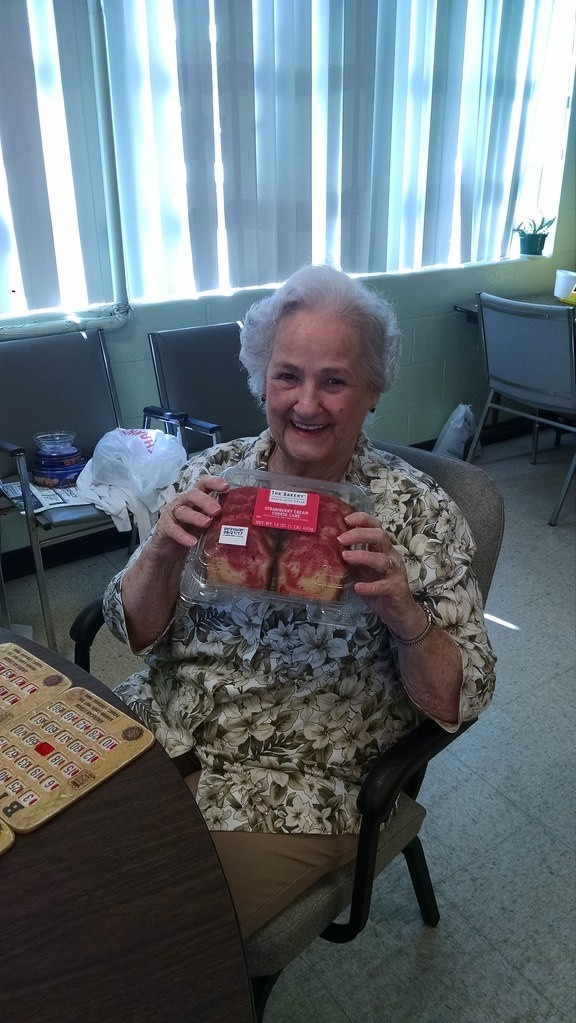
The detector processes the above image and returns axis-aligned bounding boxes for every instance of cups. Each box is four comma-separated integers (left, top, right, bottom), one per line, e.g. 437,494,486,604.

553,269,576,299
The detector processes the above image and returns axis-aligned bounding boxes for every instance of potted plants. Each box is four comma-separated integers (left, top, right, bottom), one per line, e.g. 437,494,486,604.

512,213,556,254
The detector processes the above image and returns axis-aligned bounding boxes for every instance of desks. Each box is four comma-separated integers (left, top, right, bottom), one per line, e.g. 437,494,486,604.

452,291,575,464
0,625,264,1023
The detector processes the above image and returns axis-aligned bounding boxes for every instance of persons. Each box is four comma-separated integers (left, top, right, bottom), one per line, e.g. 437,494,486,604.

103,263,497,946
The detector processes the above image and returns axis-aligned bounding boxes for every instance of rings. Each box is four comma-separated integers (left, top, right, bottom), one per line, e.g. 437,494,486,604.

378,553,395,578
170,504,186,523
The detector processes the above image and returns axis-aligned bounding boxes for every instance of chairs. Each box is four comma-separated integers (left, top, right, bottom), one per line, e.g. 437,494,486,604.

56,430,508,1023
465,293,576,526
147,318,271,459
0,326,188,655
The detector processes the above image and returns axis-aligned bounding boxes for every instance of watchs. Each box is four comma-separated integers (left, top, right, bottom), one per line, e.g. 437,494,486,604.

388,603,438,647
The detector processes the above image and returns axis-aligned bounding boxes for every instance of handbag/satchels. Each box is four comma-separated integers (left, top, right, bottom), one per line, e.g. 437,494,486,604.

434,403,481,462
93,428,187,502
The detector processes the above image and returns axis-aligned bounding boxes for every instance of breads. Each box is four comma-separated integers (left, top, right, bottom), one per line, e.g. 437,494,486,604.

205,486,359,600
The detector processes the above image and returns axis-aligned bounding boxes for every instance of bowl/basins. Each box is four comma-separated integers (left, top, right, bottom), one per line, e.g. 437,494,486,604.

32,429,76,454
177,466,376,633
